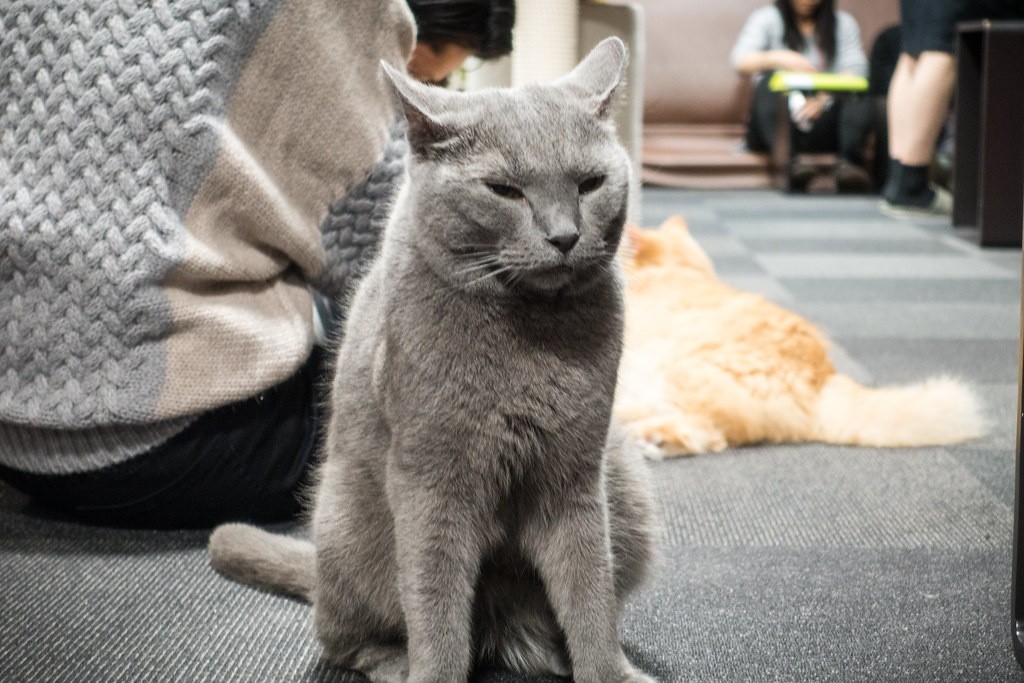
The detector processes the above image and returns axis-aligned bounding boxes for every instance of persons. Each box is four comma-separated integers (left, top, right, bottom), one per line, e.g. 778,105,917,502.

0,0,517,531
732,0,961,216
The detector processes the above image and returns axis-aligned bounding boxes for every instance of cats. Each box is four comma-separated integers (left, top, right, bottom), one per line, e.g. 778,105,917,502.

207,36,656,683
609,210,992,463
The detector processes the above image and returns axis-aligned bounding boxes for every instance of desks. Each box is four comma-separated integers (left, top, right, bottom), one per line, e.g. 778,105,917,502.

952,16,1024,247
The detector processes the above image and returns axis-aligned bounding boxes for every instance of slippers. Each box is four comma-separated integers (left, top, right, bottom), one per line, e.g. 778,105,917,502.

875,177,953,223
